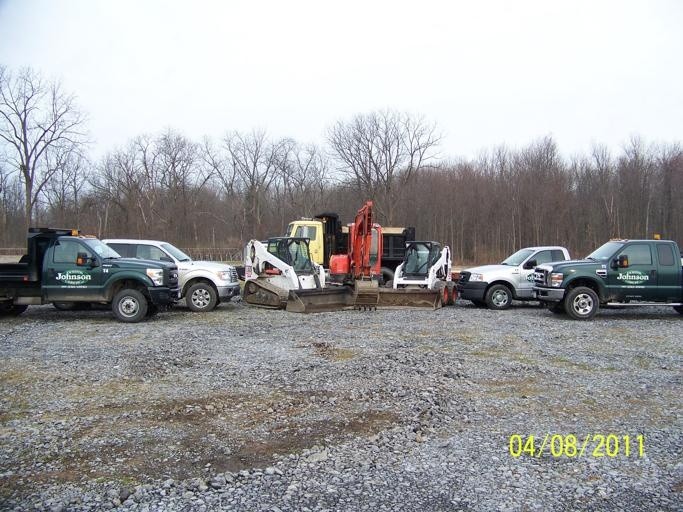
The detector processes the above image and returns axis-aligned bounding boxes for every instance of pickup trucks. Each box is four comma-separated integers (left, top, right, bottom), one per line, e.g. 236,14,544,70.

0,201,242,323
453,234,683,319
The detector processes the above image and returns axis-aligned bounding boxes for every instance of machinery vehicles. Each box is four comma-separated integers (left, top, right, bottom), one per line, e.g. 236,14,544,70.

234,201,458,314
